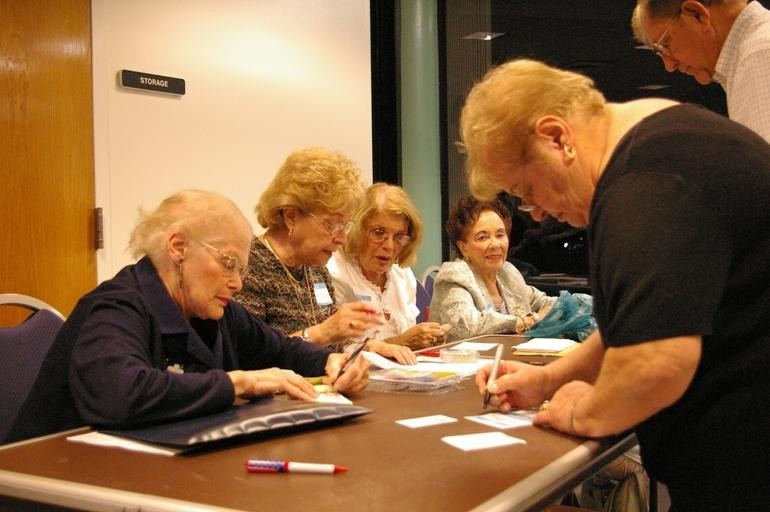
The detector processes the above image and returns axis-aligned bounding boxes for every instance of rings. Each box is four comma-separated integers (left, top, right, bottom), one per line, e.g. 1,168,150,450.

348,320,356,328
540,400,550,412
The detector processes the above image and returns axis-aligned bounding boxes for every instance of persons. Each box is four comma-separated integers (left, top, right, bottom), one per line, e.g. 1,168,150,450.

457,58,770,512
0,191,370,446
228,149,419,366
427,197,559,342
326,183,453,351
631,0,770,147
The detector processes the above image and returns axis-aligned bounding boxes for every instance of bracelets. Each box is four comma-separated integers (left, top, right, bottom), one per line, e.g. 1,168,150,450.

566,396,579,438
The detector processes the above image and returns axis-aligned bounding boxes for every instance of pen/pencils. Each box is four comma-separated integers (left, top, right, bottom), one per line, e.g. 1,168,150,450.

483,343,505,409
418,351,439,357
244,459,349,474
331,337,371,385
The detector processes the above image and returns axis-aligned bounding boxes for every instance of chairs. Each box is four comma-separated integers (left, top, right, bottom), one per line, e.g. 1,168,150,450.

417,280,433,323
0,293,68,447
421,266,441,297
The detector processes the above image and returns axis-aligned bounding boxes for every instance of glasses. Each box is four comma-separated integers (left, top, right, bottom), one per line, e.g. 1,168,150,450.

361,225,413,247
518,128,537,212
653,9,683,54
192,235,251,282
300,209,353,238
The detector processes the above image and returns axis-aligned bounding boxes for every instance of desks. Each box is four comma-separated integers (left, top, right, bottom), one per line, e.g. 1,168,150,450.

1,334,659,512
